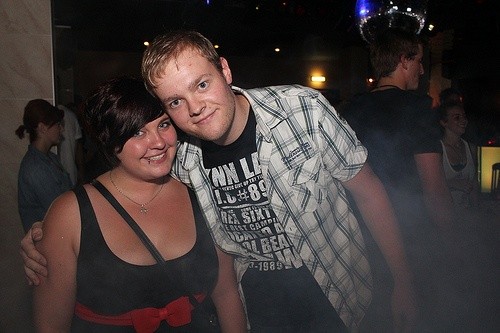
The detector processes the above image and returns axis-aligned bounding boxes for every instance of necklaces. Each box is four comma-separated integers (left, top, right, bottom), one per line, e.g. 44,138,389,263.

110,167,165,214
445,141,465,163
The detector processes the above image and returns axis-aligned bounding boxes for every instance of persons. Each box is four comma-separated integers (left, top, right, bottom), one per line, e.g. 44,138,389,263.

140,27,418,333
17,99,110,235
18,73,247,333
321,11,500,333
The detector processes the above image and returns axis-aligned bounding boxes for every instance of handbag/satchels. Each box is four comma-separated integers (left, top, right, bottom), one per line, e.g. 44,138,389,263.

193,307,220,332
